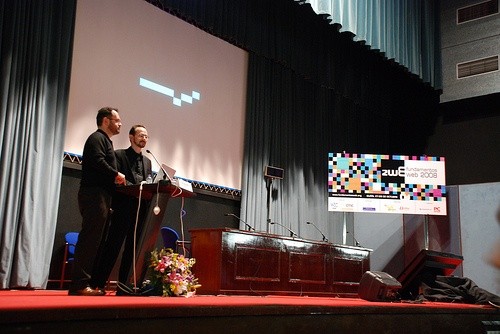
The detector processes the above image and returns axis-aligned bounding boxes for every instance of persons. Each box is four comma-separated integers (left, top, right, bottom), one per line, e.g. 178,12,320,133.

90,124,153,295
67,107,126,296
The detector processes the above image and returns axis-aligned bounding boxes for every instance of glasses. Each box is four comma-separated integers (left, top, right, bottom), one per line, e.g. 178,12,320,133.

135,135,150,140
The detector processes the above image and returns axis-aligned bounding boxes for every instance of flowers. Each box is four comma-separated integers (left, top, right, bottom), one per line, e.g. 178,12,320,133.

141,249,201,298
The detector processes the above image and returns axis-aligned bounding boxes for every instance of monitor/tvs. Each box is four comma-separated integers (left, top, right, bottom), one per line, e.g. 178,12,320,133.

395,250,465,301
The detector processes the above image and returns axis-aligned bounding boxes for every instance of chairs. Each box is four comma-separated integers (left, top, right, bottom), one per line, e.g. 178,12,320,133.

161,226,182,248
59,231,82,290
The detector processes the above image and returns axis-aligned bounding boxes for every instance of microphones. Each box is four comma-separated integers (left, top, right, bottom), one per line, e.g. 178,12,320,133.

307,222,330,242
146,150,173,185
271,222,297,236
225,213,256,231
347,232,361,247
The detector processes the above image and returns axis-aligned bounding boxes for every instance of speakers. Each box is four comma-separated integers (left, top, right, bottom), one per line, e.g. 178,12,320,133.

359,271,402,302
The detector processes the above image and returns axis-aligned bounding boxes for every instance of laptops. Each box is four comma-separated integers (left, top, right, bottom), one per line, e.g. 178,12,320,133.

152,164,176,183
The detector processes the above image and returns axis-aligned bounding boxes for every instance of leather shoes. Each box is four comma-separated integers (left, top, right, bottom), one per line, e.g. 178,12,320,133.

67,286,104,296
